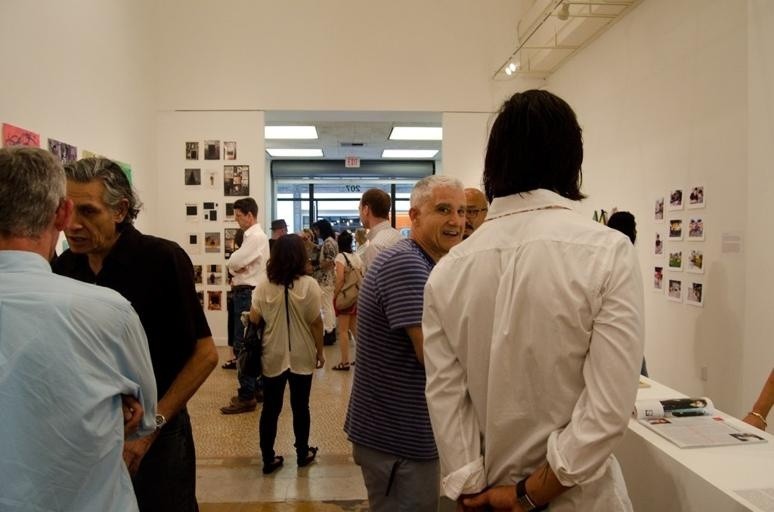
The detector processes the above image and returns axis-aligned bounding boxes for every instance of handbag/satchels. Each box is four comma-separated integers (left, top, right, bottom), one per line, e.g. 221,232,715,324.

235,316,266,378
336,251,365,310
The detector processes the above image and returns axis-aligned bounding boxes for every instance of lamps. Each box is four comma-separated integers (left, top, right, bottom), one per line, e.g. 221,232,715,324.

505,62,520,76
558,0,569,20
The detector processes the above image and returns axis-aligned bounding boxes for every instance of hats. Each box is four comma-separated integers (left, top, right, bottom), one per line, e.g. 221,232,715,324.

270,220,288,230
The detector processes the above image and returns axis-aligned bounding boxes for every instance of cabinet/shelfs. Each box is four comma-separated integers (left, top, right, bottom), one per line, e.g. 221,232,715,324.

303,216,364,251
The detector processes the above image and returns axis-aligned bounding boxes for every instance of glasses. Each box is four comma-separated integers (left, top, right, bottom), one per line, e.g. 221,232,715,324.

466,208,487,218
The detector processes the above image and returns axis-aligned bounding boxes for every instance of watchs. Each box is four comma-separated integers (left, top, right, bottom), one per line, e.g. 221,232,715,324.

151,412,166,429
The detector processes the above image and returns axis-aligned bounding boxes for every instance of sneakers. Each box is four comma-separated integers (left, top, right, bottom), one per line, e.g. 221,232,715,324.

220,389,263,414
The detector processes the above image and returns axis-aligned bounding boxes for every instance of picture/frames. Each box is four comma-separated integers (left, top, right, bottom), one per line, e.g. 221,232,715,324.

223,165,249,196
224,228,244,259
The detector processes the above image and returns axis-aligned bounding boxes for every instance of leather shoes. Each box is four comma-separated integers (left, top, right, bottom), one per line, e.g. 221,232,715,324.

264,456,283,474
297,448,316,467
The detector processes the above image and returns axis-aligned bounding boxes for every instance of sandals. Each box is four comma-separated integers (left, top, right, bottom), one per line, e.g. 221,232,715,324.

222,359,236,368
332,361,349,371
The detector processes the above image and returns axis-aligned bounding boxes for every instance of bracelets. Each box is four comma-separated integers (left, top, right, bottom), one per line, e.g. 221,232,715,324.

515,477,550,509
747,411,768,428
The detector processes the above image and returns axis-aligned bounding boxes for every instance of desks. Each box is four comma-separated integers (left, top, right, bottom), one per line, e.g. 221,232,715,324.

612,374,774,512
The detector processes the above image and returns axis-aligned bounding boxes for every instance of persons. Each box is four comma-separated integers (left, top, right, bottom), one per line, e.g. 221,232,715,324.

0,147,153,511
311,189,405,372
604,210,649,378
51,157,220,511
460,188,488,238
424,88,650,512
342,171,469,512
663,395,708,411
739,367,774,431
221,197,327,479
